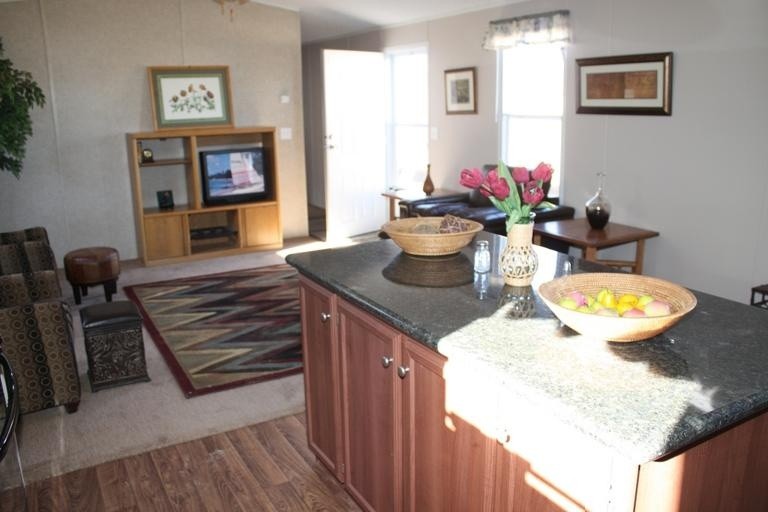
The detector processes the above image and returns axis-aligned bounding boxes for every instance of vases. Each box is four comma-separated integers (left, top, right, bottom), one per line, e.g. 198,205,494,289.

497,221,536,287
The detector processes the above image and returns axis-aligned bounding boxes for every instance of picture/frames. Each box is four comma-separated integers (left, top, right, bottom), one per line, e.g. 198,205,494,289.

443,66,476,115
573,51,671,118
145,63,234,132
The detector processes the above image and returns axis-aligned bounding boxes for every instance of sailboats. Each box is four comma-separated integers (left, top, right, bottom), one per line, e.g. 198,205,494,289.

227,152,262,189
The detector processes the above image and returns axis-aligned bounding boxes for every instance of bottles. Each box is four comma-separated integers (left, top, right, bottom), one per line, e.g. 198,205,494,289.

585,187,612,230
471,274,491,300
472,240,491,273
423,164,434,194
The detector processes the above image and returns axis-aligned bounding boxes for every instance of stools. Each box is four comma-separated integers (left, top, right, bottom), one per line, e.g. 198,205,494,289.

64,246,121,305
79,299,150,393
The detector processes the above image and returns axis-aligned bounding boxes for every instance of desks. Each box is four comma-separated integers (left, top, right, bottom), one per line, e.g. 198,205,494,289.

531,217,660,273
398,194,576,256
285,221,768,512
379,187,460,221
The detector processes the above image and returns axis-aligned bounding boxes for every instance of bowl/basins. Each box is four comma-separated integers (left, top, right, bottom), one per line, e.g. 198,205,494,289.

537,272,698,342
381,250,475,287
384,216,485,256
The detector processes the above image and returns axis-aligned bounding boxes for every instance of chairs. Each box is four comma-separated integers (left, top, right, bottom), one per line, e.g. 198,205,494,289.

0,270,80,417
0,227,57,276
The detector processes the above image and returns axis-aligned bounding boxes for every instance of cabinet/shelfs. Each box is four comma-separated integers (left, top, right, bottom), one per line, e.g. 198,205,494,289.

125,126,282,268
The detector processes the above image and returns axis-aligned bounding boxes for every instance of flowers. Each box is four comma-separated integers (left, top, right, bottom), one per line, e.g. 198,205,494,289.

457,164,553,228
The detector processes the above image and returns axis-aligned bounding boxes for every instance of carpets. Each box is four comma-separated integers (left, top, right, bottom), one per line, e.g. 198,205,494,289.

0,230,398,494
121,261,301,401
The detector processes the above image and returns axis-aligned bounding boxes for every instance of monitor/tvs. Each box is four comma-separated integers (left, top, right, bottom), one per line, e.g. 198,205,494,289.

199,146,267,205
